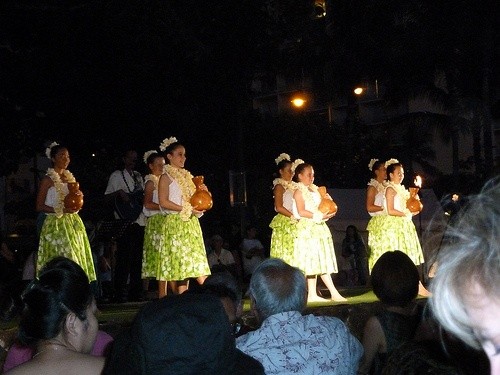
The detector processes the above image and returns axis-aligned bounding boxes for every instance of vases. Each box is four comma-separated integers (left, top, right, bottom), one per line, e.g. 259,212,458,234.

318,186,338,214
65,181,84,213
407,188,424,212
191,175,213,210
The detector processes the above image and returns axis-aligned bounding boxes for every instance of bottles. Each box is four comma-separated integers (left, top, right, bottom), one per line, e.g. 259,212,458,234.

406,188,423,212
190,176,213,211
64,183,83,211
317,186,337,215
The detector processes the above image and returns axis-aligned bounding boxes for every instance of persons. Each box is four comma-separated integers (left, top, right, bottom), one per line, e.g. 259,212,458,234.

201,285,249,337
358,251,429,375
0,267,111,375
242,227,266,297
4,257,113,371
350,241,365,287
422,177,500,375
204,272,244,317
158,137,212,293
36,142,100,316
102,291,264,375
142,150,178,298
342,225,368,283
346,307,369,341
235,258,365,375
105,150,147,302
380,158,431,297
382,341,469,375
290,158,347,302
208,235,236,270
304,301,365,322
0,152,140,297
201,237,233,254
0,273,15,375
366,158,389,276
270,153,299,266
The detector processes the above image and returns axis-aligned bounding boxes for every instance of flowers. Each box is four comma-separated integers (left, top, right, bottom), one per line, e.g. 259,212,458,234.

274,153,291,166
384,159,398,168
290,158,304,171
159,137,177,150
163,164,196,200
144,173,160,188
273,178,297,189
368,178,384,202
314,210,325,224
143,150,157,163
404,211,415,223
45,142,58,158
179,202,193,221
368,158,379,172
287,181,332,209
47,168,80,203
385,181,409,210
53,203,64,220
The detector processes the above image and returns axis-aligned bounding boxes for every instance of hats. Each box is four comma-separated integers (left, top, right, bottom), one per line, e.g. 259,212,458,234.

211,234,223,240
